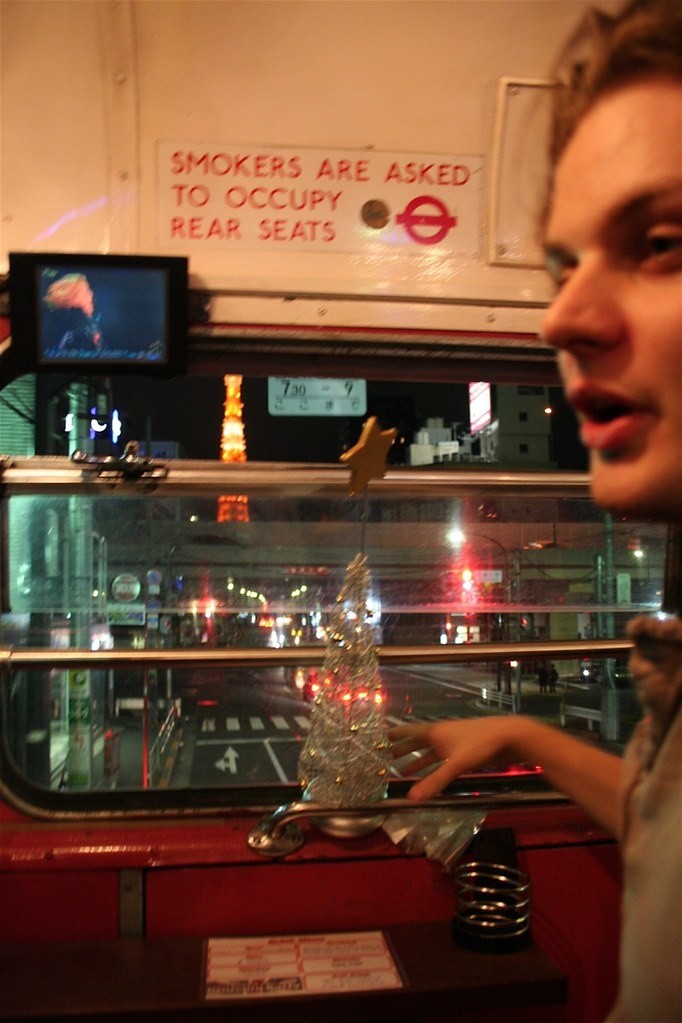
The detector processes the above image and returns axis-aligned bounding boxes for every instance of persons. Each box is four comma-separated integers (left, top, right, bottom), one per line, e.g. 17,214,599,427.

384,3,682,1023
43,271,104,350
537,662,559,693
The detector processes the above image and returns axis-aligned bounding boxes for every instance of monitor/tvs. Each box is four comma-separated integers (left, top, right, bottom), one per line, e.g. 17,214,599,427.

9,253,190,374
